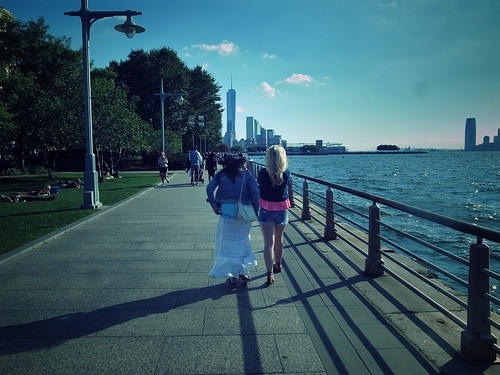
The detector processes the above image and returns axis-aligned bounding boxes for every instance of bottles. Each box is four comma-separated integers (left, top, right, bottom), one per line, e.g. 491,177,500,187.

15,196,19,203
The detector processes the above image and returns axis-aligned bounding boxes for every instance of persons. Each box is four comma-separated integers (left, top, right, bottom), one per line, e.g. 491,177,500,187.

256,144,294,284
200,151,212,170
206,153,261,282
188,150,192,172
32,185,51,195
216,151,244,169
190,145,202,186
112,171,124,179
57,177,82,188
104,172,114,179
206,152,216,182
159,152,170,187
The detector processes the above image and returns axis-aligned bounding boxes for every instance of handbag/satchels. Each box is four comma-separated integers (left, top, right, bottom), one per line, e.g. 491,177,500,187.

238,202,257,222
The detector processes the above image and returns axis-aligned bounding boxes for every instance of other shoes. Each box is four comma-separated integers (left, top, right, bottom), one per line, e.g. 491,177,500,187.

273,262,281,272
166,178,169,183
267,271,274,284
229,275,237,284
239,275,250,282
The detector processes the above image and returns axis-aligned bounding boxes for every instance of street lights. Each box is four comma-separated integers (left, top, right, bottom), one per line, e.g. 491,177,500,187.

153,79,189,152
64,0,147,208
